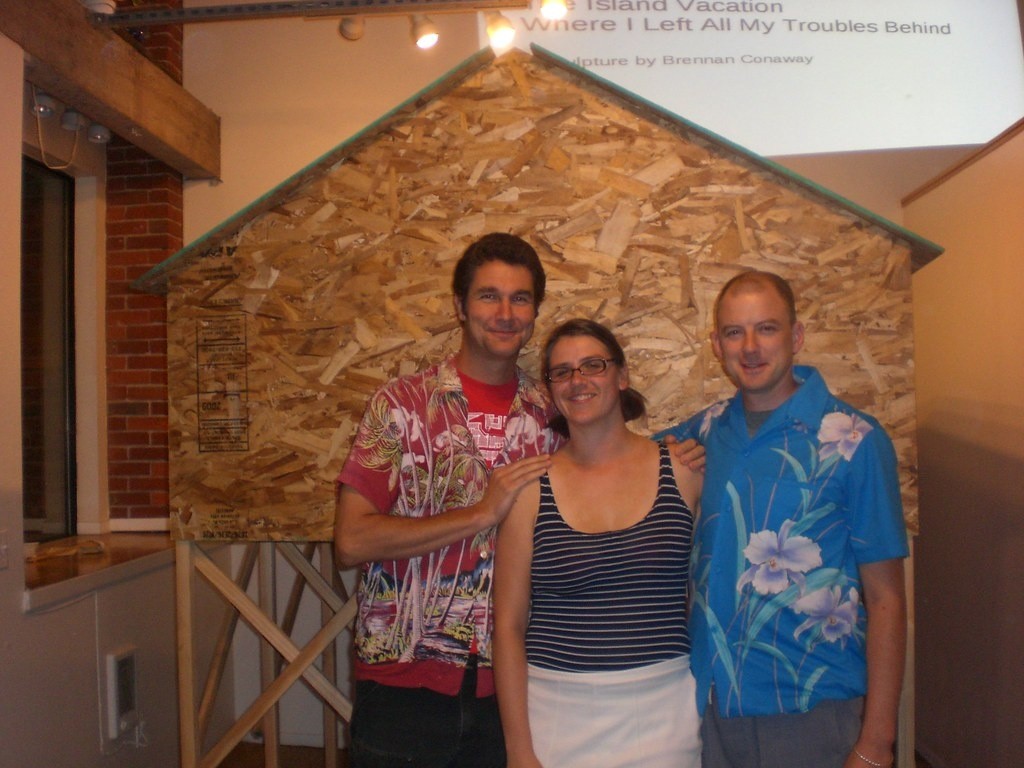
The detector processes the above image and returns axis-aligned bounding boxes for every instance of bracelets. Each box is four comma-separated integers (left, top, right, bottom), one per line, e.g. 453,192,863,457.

853,744,894,767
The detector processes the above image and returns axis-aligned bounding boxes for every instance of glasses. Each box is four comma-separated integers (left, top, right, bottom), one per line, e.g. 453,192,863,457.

545,357,616,382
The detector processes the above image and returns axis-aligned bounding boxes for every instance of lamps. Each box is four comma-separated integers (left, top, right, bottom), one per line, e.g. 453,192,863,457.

483,11,515,44
410,15,439,49
338,18,364,41
540,0,568,17
88,122,110,143
60,108,83,131
30,88,54,118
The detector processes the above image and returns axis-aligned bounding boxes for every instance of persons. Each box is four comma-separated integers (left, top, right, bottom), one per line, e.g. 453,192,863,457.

333,232,705,768
493,315,704,768
646,271,910,768
